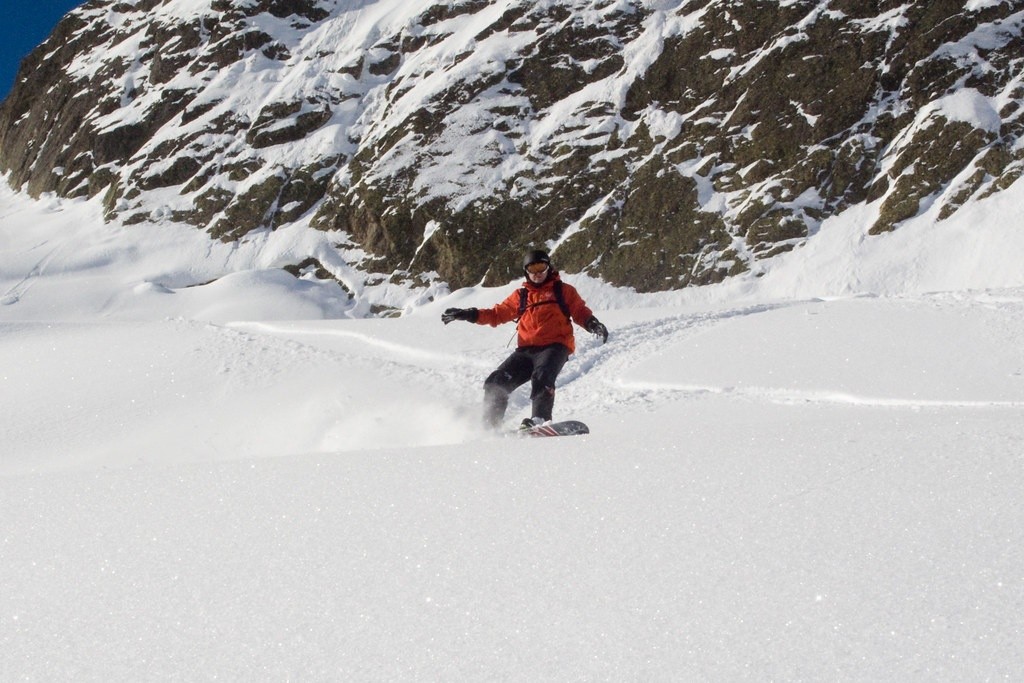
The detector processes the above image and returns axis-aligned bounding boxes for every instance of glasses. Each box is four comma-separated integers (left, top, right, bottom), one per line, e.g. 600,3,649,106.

526,265,549,276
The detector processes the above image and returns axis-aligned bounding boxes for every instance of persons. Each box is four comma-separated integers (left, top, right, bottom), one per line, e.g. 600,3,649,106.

441,251,609,428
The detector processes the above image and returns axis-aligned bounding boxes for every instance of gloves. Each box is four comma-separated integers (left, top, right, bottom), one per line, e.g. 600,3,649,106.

584,316,608,344
441,307,477,325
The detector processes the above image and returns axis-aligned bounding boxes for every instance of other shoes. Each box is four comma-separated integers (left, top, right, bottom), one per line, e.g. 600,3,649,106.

522,418,537,428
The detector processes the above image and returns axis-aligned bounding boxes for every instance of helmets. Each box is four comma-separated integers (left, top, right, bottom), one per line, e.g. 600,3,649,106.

523,250,550,268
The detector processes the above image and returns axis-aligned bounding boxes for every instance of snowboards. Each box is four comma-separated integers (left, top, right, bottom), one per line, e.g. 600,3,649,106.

517,419,590,437
